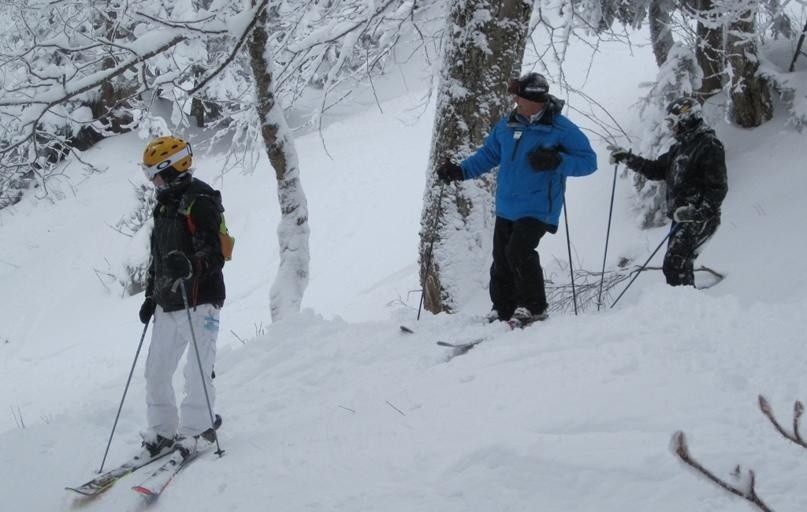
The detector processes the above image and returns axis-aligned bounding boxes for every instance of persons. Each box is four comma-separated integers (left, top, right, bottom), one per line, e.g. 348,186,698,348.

434,72,598,328
135,135,227,460
604,96,729,287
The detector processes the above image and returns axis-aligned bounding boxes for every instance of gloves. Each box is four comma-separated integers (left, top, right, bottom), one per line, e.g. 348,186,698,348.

163,254,187,278
139,295,154,324
436,160,461,184
532,146,558,172
607,144,637,168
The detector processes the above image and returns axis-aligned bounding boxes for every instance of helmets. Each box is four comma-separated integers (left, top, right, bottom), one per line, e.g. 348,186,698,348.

144,136,193,172
664,97,703,130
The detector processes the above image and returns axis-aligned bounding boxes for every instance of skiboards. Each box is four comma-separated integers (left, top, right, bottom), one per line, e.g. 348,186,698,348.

400,304,548,347
64,415,222,496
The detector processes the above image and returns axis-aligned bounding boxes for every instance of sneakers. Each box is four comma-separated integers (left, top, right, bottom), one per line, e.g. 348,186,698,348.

141,431,216,457
489,304,532,318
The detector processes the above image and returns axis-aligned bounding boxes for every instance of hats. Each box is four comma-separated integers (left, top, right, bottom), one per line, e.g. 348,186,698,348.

508,72,549,102
